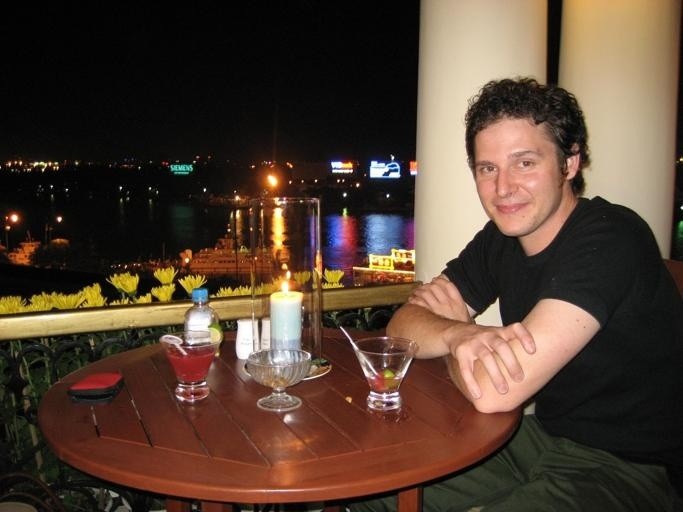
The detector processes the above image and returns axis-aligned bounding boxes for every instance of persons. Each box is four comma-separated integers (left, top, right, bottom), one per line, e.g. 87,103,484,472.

343,72,683,510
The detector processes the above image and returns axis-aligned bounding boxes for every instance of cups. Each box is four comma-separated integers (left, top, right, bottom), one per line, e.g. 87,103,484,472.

159,330,222,402
248,194,323,371
353,337,418,413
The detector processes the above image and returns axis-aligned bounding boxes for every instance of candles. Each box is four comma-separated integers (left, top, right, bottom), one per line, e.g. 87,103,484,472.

270,280,303,349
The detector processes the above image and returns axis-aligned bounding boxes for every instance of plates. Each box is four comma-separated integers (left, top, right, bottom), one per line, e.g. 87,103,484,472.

241,357,332,382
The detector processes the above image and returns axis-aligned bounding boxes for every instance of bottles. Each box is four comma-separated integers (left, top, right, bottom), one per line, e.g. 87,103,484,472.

262,316,271,349
184,288,220,357
235,318,260,361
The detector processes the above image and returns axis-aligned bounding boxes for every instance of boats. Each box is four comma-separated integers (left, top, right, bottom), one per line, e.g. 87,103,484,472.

5,232,70,271
347,245,415,283
103,255,180,276
179,238,291,283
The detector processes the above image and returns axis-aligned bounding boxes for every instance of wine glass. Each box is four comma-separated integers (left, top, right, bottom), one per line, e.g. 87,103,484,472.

249,348,311,413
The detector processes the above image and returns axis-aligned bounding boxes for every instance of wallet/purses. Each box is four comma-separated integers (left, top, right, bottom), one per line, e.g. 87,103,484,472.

66,372,123,405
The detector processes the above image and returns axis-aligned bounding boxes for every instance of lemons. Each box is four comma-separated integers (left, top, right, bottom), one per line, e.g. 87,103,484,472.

208,325,223,347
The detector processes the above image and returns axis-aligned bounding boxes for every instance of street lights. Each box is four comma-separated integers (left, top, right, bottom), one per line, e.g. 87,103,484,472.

43,216,64,250
257,175,278,285
2,215,11,253
229,194,241,285
